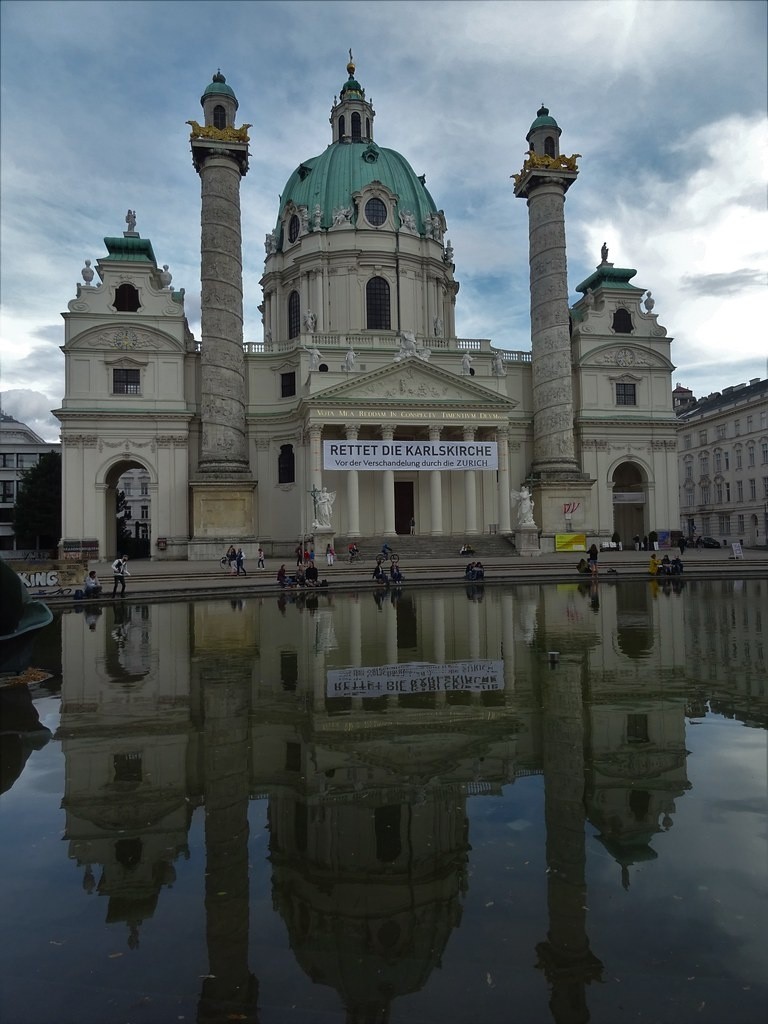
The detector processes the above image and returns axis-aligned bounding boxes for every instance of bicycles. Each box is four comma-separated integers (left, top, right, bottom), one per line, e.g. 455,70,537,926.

376,551,399,564
220,557,232,569
343,550,365,565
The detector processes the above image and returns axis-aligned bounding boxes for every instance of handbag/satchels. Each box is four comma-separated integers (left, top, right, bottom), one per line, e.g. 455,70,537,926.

330,547,334,555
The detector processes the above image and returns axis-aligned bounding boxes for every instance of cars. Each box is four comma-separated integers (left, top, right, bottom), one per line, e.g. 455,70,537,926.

701,536,720,548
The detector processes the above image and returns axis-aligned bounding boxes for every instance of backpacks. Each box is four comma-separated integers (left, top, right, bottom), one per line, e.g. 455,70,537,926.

305,551,310,560
348,544,353,550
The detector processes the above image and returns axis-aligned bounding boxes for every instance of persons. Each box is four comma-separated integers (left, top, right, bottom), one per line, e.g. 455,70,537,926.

85,555,130,598
85,602,130,648
127,927,143,952
230,586,402,617
586,530,705,577
621,867,631,894
126,209,136,232
459,543,477,557
315,487,337,527
589,579,687,615
264,204,454,263
227,542,406,589
466,584,485,604
409,516,415,536
601,242,609,262
511,486,534,524
465,561,485,581
264,309,507,376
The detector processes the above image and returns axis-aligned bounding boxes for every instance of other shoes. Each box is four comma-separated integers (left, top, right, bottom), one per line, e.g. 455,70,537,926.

244,571,246,576
121,594,125,598
261,568,265,570
328,564,330,566
257,568,260,569
330,564,332,566
349,561,352,564
111,595,115,598
